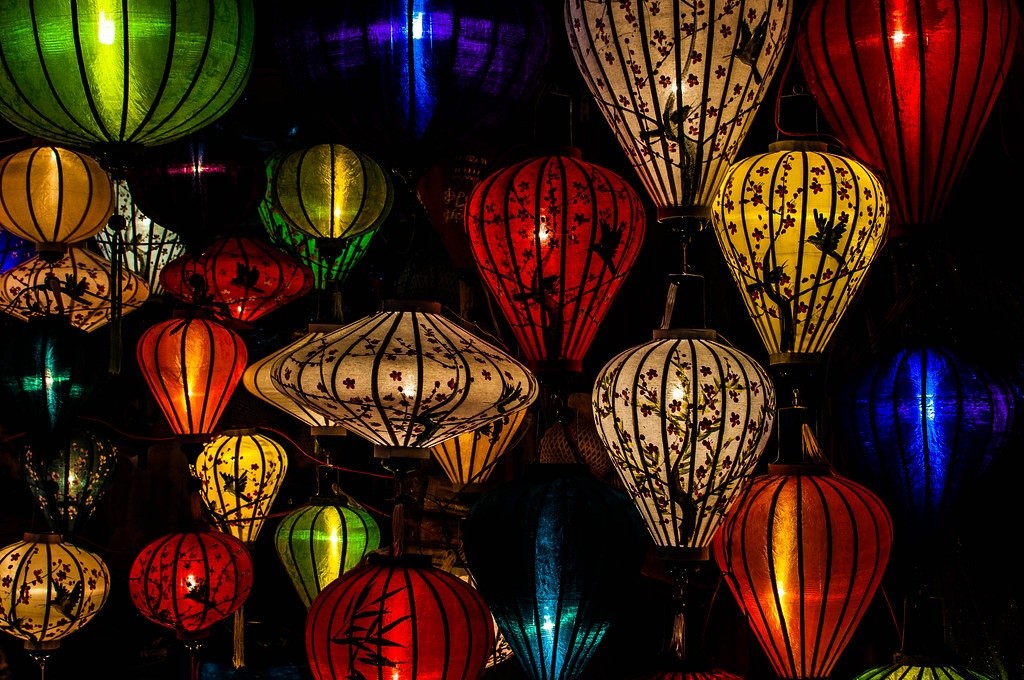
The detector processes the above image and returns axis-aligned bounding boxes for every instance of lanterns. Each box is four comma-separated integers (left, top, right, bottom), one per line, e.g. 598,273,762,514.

0,0,1024,680
